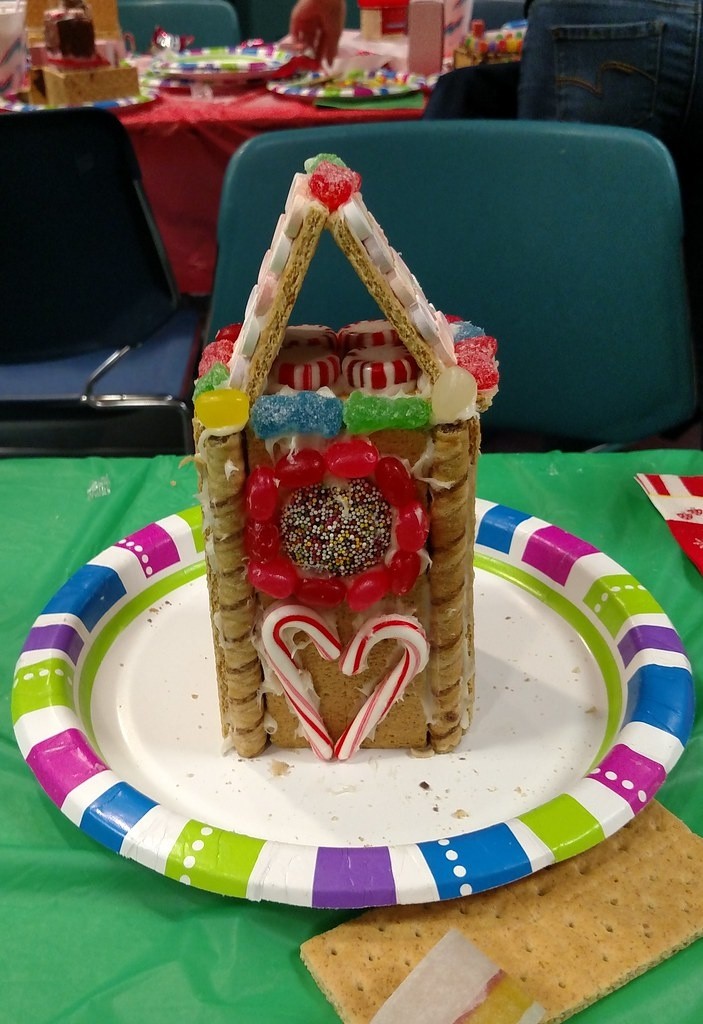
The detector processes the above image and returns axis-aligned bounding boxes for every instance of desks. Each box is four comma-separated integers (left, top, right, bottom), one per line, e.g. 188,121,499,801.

122,94,434,295
0,449,703,1024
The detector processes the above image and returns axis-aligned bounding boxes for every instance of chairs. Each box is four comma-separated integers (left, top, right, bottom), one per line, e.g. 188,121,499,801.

115,0,238,54
203,119,699,451
0,107,203,456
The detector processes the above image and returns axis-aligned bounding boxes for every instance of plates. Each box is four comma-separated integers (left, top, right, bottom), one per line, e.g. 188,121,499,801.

267,72,432,97
10,487,697,907
150,46,293,76
2,82,159,108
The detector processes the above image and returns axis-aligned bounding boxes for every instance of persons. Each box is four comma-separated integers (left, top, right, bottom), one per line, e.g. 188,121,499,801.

289,0,703,205
